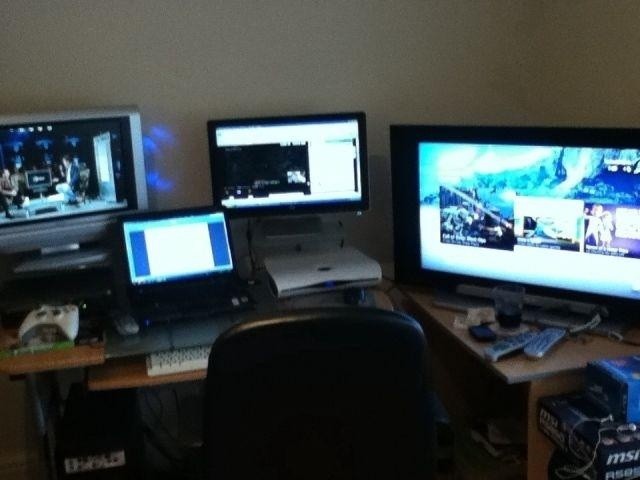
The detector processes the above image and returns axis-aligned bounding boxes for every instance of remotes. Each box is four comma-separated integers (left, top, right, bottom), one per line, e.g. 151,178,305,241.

483,330,540,361
523,327,568,362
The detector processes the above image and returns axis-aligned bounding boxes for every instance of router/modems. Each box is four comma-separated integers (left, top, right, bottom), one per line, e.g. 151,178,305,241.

261,245,384,300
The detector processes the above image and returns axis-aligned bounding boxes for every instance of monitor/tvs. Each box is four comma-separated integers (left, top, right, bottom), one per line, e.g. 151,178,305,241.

205,110,370,238
0,104,152,275
389,123,640,344
115,202,238,290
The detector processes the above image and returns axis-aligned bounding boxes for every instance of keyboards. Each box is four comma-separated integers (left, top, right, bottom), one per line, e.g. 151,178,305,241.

142,344,212,378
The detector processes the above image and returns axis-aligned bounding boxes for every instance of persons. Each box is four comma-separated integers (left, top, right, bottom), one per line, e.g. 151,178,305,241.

583,203,615,250
0,165,25,220
52,155,83,207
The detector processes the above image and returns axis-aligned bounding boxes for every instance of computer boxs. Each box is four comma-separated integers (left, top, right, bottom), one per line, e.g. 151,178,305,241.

52,380,151,480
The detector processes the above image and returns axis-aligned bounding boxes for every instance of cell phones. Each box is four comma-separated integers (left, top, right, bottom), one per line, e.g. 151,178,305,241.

469,323,499,342
